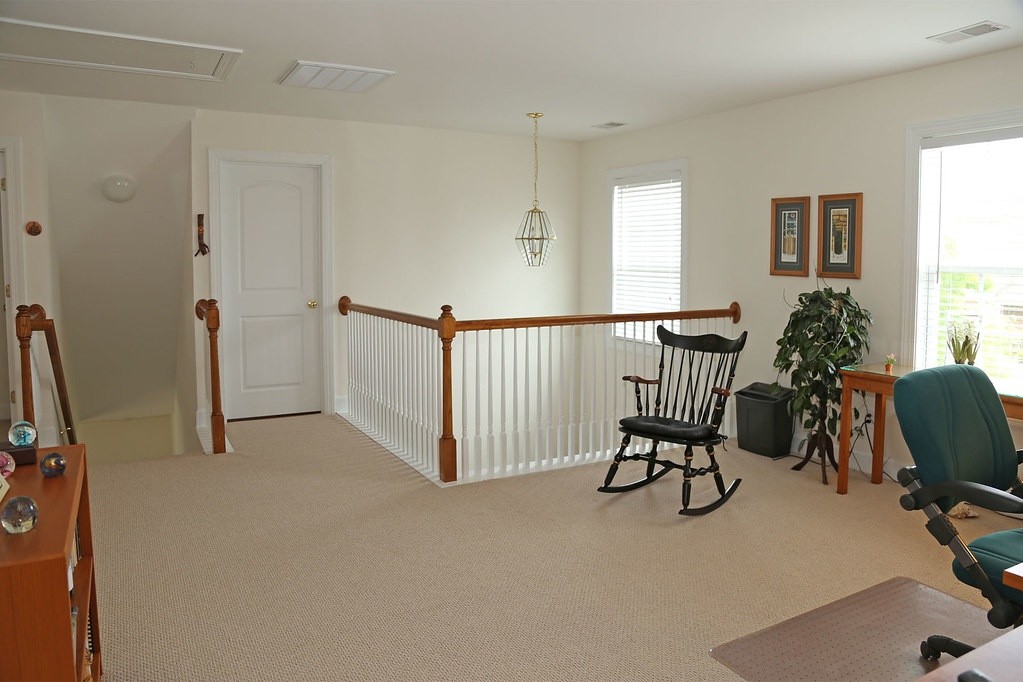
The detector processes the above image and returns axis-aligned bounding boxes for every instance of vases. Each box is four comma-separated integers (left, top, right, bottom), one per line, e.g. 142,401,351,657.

885,364,893,371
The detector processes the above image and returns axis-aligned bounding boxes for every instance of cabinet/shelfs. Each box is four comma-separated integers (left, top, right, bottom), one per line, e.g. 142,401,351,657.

0,444,103,682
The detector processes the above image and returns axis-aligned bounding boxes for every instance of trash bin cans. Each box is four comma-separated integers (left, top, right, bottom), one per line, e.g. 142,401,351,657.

733,381,797,458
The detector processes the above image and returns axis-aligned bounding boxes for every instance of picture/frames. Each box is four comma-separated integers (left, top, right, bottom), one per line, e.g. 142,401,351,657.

817,193,863,280
770,197,810,276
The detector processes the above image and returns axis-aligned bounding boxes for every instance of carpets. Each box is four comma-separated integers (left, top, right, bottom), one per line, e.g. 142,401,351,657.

708,575,1014,682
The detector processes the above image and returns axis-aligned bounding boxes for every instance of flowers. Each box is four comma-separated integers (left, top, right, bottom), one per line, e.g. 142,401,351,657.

885,354,896,364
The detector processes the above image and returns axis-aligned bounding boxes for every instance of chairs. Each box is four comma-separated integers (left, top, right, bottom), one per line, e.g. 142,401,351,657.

598,325,747,515
893,364,1023,659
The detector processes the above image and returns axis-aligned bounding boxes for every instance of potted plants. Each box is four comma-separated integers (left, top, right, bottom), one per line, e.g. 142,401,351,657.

966,332,980,365
772,268,872,457
947,328,975,364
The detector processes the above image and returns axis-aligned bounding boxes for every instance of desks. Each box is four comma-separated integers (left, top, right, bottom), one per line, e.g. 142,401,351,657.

837,362,1023,494
1003,561,1023,591
914,624,1023,682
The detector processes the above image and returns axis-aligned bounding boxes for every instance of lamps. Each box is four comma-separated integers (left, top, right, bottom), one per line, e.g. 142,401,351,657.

104,172,138,203
515,113,556,267
